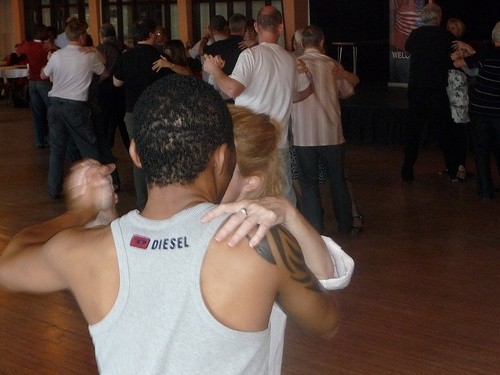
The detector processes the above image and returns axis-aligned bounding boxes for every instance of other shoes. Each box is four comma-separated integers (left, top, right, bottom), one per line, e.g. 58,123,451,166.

35,143,44,148
48,193,59,198
402,166,415,182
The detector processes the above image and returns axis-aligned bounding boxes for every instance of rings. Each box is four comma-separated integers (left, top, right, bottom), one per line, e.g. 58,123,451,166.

239,207,249,219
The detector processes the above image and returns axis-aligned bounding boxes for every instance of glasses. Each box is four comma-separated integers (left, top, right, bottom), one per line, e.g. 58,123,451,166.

154,32,161,36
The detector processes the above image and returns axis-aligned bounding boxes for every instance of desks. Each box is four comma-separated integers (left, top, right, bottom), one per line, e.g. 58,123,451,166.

0,66,28,108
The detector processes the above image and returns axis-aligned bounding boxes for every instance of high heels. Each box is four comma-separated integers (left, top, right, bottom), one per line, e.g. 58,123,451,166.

352,215,366,234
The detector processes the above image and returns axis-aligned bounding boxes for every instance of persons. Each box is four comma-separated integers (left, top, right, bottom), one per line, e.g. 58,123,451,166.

292,25,355,239
65,104,358,373
1,73,341,374
288,31,366,235
402,4,453,193
442,16,479,183
5,4,258,201
201,5,299,207
454,21,500,207
